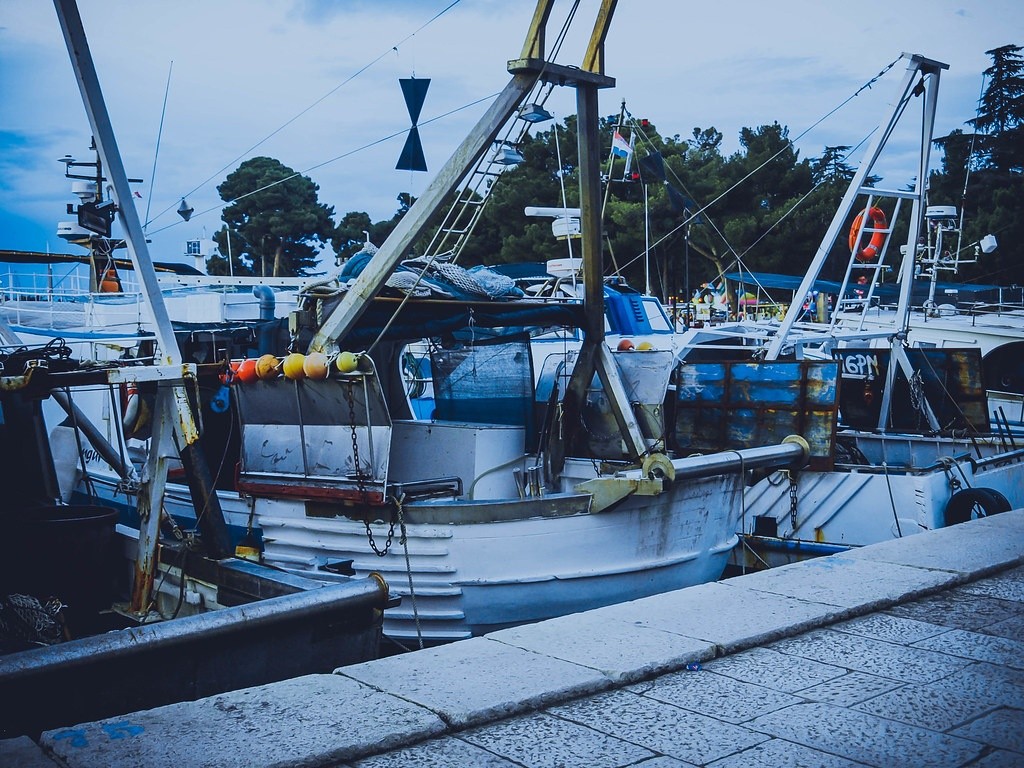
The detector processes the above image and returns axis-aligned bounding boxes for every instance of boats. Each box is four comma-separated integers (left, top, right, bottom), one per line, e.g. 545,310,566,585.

0,0,1023,740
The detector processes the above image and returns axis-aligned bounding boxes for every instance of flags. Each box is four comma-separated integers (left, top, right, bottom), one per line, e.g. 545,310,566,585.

612,131,633,159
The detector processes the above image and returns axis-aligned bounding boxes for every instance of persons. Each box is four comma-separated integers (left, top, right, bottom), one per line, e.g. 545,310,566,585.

123,381,151,436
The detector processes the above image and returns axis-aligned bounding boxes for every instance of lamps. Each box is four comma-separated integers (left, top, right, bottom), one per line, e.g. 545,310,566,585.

77,199,117,238
516,102,553,123
488,141,524,164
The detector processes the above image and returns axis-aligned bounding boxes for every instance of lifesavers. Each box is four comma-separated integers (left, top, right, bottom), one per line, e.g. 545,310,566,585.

848,206,887,261
